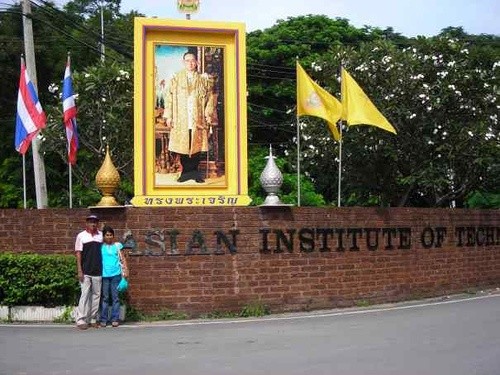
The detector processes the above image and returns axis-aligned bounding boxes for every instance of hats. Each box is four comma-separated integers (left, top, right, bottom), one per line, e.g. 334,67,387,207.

85,214,99,221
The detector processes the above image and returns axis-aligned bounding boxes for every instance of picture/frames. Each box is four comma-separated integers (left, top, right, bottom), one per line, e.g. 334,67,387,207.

129,16,254,207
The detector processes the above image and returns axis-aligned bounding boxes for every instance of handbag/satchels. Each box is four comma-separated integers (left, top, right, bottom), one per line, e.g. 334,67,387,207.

117,277,128,293
119,262,126,276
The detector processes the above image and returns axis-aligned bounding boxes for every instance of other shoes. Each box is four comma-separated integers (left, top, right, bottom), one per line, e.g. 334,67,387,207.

77,324,88,329
89,322,100,328
177,175,190,182
112,322,118,327
192,177,205,183
100,322,106,326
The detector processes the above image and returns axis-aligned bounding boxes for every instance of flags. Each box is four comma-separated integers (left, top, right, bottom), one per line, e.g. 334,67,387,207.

15,56,47,153
340,68,397,135
296,62,343,141
64,56,78,165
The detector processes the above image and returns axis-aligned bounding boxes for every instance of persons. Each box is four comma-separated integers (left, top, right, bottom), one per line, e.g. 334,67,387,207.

75,215,103,330
100,227,128,327
164,52,219,183
